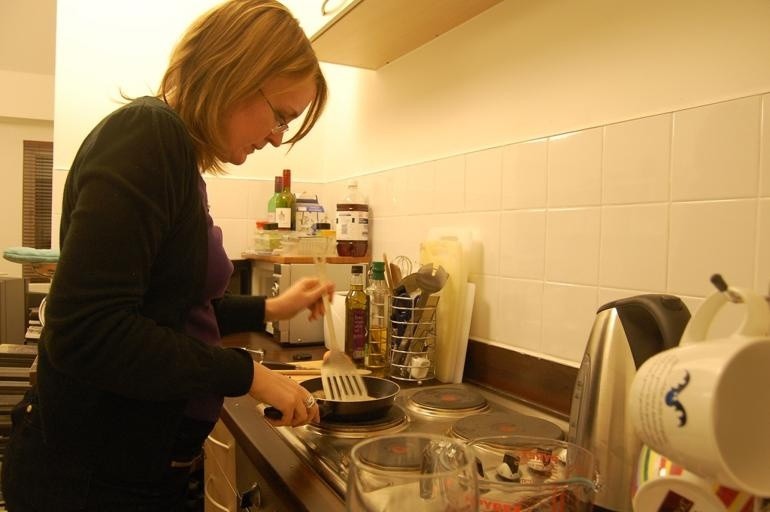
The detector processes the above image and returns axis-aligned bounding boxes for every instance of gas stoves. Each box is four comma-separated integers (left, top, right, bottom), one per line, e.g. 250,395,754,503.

257,374,571,499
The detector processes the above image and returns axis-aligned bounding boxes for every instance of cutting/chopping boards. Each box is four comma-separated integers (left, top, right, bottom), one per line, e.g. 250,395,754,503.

418,239,476,385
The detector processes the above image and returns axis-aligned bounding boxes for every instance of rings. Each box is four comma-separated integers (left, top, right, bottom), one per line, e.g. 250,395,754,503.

304,395,315,408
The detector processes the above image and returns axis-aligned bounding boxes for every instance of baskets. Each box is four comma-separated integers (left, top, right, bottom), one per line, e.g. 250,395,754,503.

389,295,440,385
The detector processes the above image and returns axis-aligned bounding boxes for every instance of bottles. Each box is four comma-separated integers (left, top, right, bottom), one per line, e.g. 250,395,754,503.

345,265,370,365
336,179,369,256
314,222,336,256
268,168,296,231
254,222,279,251
367,261,391,369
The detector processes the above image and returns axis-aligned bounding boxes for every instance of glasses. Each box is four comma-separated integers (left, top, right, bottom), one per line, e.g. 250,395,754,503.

259,89,289,136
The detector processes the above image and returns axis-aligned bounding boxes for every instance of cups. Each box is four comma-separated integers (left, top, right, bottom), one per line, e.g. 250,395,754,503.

628,287,770,512
347,433,479,512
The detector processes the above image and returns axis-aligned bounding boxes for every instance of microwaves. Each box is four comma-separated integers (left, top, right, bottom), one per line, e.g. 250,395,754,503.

224,260,371,344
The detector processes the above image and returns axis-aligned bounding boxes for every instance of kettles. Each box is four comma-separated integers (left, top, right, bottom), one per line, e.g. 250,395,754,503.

564,294,692,512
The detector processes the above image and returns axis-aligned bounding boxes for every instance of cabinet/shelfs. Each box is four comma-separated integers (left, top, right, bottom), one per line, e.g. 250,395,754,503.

287,0,504,70
203,418,289,512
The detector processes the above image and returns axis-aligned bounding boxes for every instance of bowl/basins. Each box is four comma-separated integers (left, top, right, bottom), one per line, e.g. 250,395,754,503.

456,437,599,511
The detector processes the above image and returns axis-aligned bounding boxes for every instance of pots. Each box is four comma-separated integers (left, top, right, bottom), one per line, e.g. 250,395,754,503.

264,376,400,421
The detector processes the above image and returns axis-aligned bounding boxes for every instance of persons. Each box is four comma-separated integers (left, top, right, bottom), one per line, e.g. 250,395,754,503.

0,1,335,512
